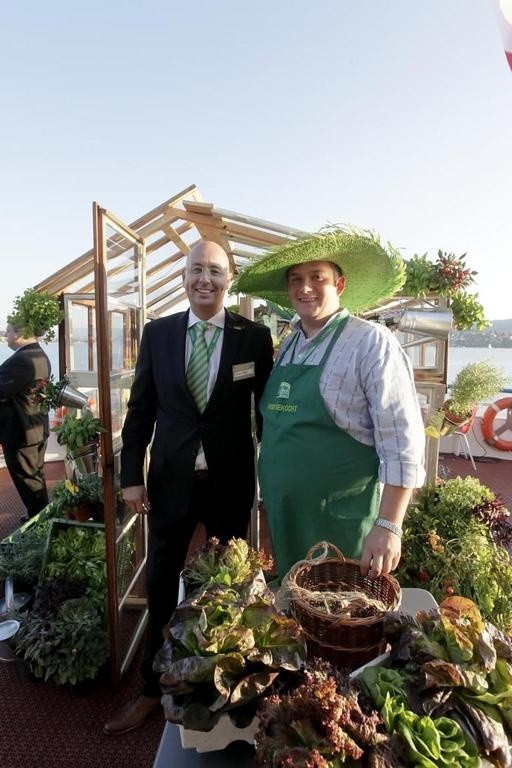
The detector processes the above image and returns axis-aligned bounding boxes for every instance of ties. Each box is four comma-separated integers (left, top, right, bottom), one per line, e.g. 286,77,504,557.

184,321,210,413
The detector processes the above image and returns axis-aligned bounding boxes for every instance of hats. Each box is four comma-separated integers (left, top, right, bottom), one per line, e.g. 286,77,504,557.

228,221,408,315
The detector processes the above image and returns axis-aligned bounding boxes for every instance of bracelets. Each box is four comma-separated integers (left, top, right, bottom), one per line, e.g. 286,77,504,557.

373,515,405,539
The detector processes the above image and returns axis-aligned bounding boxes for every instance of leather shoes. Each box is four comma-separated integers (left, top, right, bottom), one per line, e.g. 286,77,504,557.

101,693,162,736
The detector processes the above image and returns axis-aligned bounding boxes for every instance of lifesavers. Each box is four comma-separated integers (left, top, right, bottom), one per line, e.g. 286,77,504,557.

482,397,511,452
54,397,99,427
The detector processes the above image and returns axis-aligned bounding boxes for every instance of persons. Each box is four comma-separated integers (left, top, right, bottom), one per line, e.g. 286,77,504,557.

98,241,272,736
228,228,427,587
0,310,52,524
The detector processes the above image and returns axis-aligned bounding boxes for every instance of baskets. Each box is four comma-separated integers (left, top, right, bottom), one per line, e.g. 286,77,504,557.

288,541,403,651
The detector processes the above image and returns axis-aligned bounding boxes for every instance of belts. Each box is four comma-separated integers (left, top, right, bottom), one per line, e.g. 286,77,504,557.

194,470,209,482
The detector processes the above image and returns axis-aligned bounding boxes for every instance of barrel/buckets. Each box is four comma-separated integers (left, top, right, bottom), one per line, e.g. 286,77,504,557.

70,441,98,476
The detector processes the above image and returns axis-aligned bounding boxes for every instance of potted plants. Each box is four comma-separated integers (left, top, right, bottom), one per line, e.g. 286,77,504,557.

54,481,125,521
397,294,491,341
154,539,308,754
29,374,87,412
56,417,105,477
442,361,509,434
